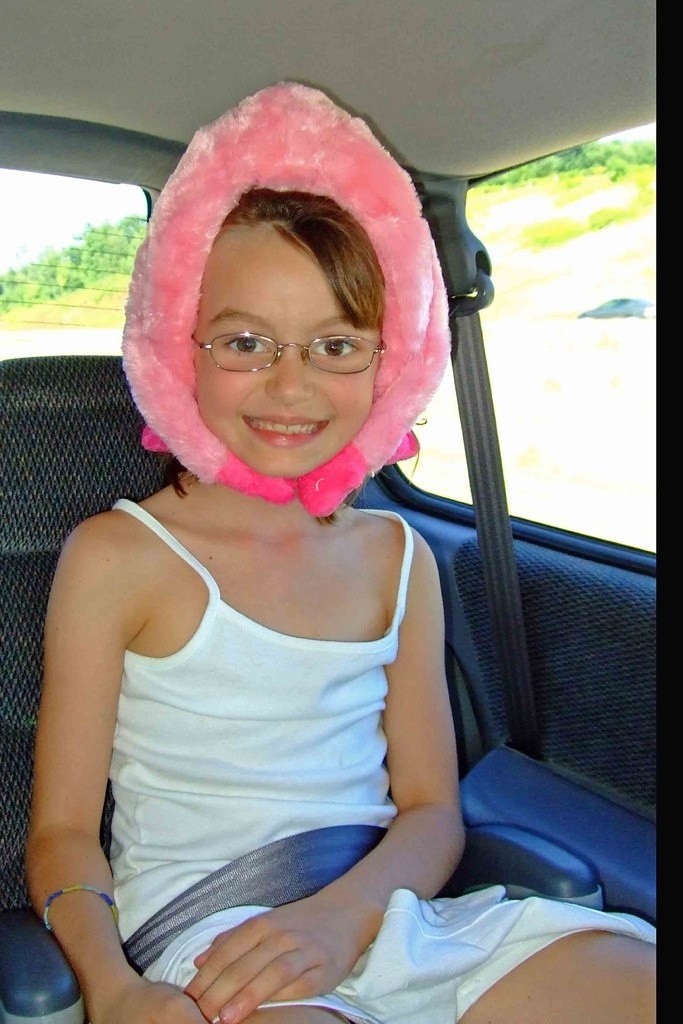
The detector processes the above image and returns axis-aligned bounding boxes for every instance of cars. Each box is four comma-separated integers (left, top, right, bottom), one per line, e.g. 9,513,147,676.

576,297,654,319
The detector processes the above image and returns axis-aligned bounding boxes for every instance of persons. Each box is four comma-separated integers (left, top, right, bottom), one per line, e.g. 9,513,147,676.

25,187,656,1024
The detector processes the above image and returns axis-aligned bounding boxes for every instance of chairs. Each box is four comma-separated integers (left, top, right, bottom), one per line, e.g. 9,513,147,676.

0,355,602,1024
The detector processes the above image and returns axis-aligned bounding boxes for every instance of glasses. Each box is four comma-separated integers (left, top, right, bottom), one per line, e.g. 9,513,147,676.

192,330,387,375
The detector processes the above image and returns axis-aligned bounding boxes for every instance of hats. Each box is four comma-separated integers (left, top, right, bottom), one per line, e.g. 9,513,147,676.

119,78,452,516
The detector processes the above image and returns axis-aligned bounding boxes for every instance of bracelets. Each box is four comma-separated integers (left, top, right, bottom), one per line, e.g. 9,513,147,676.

44,885,118,931
